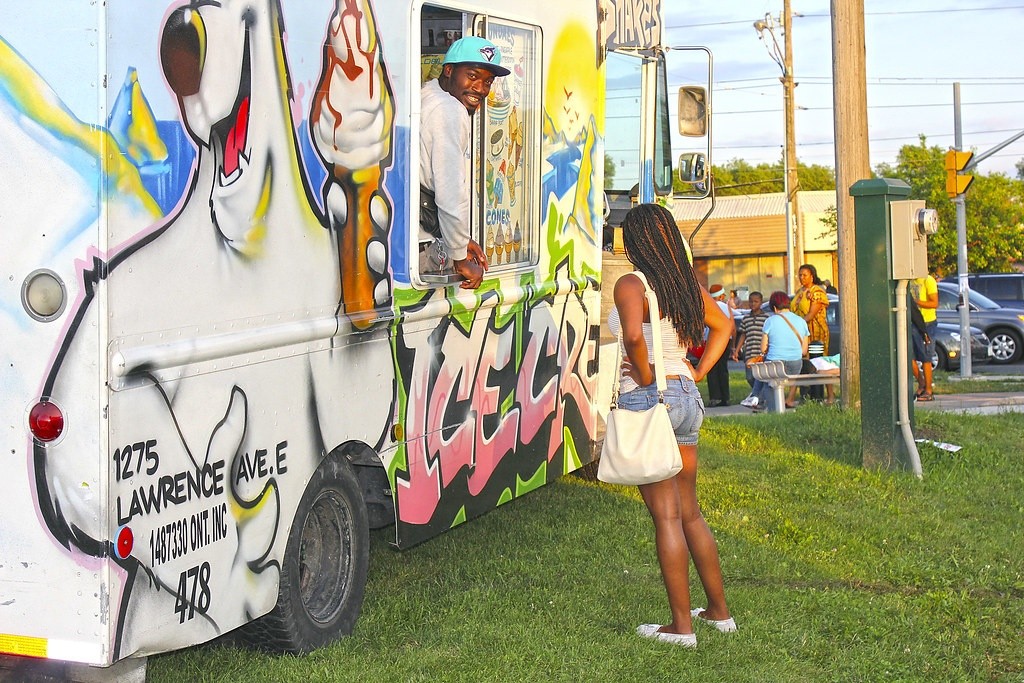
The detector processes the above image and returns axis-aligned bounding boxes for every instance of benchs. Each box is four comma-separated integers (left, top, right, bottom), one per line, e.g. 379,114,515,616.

748,361,840,414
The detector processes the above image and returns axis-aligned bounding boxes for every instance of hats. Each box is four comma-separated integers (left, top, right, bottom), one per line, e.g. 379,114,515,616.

443,37,512,77
710,284,725,297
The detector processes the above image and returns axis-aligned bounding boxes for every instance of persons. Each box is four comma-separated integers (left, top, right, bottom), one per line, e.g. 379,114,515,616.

419,36,511,290
816,277,838,321
607,203,737,647
727,288,743,309
732,291,770,388
740,291,809,414
909,276,939,401
701,283,737,407
785,264,835,408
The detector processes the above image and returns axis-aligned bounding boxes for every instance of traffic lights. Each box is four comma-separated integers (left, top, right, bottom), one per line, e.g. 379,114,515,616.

945,149,976,199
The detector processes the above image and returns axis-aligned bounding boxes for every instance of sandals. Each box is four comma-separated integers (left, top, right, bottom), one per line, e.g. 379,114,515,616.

914,391,935,401
913,390,925,399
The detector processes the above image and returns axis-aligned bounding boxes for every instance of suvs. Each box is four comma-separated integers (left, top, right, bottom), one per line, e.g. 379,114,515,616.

935,272,1023,311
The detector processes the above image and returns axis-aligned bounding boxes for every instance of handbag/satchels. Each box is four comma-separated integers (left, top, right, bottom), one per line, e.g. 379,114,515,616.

745,354,764,369
420,184,443,237
596,401,683,486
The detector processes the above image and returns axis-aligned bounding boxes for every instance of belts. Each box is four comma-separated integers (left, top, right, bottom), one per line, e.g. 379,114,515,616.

419,241,431,253
666,375,691,380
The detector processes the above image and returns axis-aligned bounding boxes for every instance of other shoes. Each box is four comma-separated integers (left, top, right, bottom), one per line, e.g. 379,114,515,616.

637,624,696,649
786,403,795,408
740,392,758,407
691,608,737,633
717,401,729,406
707,399,721,406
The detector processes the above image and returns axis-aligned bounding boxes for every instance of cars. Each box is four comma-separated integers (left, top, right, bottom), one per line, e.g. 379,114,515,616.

932,282,1023,363
703,301,748,363
742,291,839,319
823,299,991,373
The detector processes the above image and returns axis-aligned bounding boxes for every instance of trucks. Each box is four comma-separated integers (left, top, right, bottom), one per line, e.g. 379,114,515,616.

0,1,716,674
